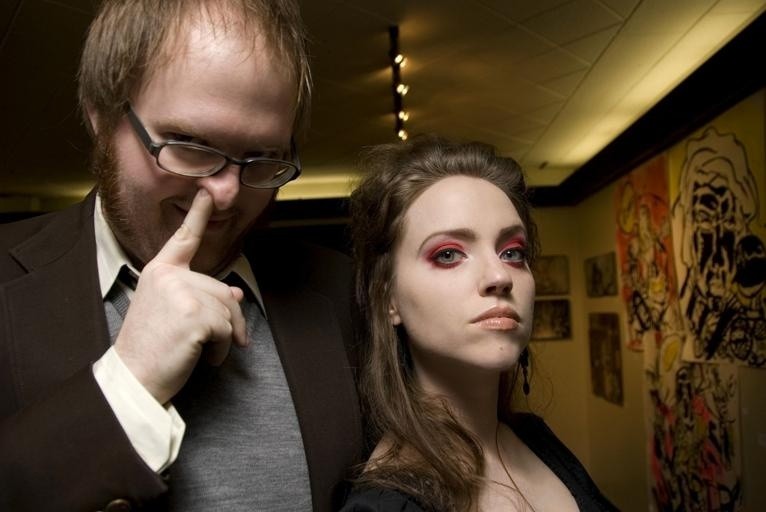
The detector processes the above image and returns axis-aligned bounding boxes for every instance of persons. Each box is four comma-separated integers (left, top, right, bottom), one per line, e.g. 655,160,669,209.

335,137,621,511
0,0,378,512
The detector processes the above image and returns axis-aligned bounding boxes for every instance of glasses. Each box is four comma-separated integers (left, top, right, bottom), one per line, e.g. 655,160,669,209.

124,102,304,193
